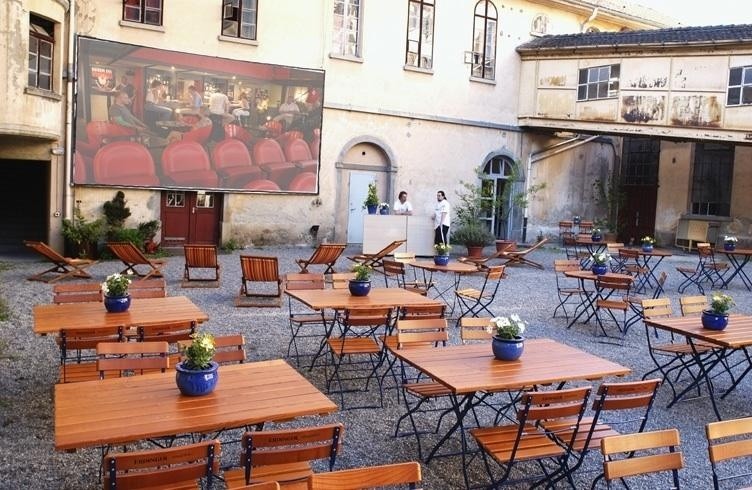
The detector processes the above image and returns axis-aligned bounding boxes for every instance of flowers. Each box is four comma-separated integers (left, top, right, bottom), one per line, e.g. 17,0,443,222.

379,201,390,209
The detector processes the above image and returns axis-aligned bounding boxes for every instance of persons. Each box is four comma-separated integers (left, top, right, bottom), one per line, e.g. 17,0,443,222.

429,189,450,248
108,76,321,133
393,190,413,215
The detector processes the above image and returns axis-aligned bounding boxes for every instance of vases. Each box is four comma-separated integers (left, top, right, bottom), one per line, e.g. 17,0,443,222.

380,208,390,215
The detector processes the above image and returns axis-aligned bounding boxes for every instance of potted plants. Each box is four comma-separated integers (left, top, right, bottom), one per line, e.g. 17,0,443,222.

452,159,548,257
361,183,379,214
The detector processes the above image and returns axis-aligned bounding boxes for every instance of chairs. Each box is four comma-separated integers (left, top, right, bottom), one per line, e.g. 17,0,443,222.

74,108,321,193
